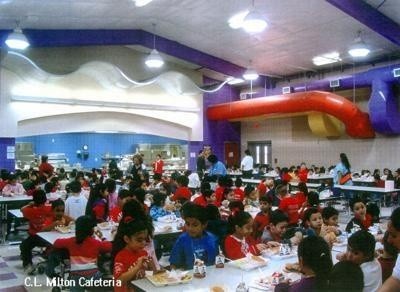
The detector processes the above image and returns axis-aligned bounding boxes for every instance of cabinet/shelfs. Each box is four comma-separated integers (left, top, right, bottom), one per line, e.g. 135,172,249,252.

138,142,186,164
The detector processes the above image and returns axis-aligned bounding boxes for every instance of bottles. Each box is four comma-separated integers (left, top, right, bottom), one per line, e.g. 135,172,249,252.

236,281,250,292
192,260,206,277
271,242,290,286
215,251,225,268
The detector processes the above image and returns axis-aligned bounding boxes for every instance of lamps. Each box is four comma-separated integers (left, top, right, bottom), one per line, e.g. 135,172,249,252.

145,23,165,70
346,28,370,58
242,58,260,83
4,18,30,51
229,2,271,34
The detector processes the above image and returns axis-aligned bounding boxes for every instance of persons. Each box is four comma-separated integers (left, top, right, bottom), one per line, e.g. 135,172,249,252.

1,149,400,291
